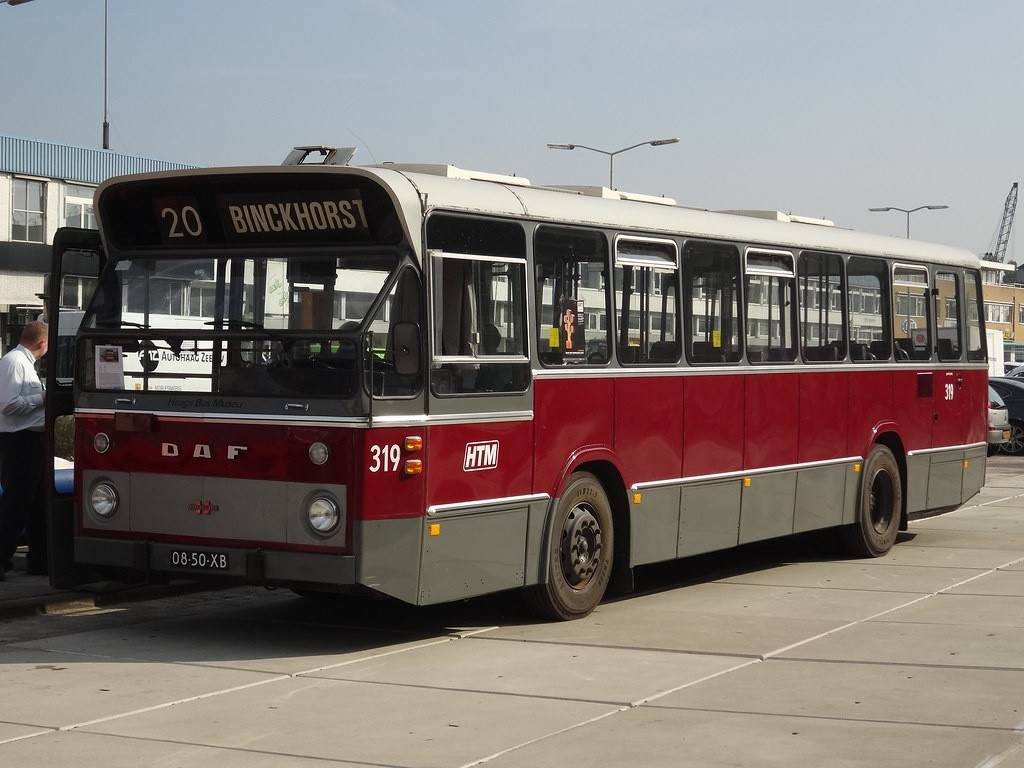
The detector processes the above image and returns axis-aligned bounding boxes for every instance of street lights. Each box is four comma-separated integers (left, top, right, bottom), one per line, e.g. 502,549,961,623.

868,204,950,239
547,138,683,190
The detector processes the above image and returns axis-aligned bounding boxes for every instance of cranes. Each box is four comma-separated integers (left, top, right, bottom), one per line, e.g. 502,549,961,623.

980,182,1018,262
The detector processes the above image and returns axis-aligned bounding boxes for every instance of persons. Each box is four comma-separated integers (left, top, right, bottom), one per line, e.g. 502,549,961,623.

335,320,379,359
0,321,49,580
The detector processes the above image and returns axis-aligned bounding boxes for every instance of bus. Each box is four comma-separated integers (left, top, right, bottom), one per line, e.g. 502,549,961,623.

42,145,990,622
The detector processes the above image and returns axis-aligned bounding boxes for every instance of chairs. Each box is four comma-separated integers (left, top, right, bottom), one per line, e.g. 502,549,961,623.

216,338,955,394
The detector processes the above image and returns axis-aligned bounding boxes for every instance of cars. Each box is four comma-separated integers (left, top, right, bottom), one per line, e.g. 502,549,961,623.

987,361,1024,457
